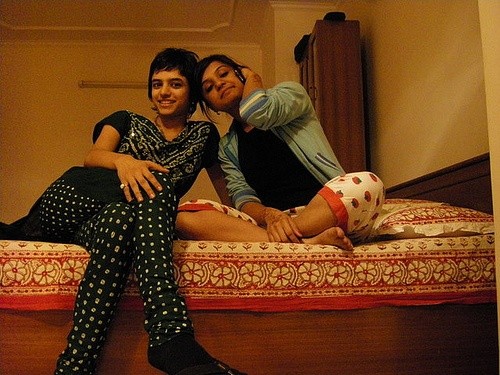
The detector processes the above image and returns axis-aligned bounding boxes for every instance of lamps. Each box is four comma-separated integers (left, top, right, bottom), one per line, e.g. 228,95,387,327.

78,80,148,89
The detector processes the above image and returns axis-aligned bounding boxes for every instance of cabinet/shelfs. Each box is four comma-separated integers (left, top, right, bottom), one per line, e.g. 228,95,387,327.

299,20,367,173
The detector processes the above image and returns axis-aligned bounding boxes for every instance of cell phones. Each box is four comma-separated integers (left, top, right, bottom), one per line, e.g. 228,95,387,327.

234,69,244,81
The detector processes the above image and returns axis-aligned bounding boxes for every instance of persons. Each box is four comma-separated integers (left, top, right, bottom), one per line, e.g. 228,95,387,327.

24,48,248,375
175,54,385,251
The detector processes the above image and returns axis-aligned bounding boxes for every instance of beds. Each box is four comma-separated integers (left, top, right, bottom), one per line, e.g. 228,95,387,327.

0,152,500,375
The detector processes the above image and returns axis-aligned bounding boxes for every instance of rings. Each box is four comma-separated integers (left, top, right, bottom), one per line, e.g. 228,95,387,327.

120,184,127,189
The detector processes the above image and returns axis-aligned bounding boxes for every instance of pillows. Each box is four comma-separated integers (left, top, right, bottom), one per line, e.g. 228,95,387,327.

368,199,495,240
